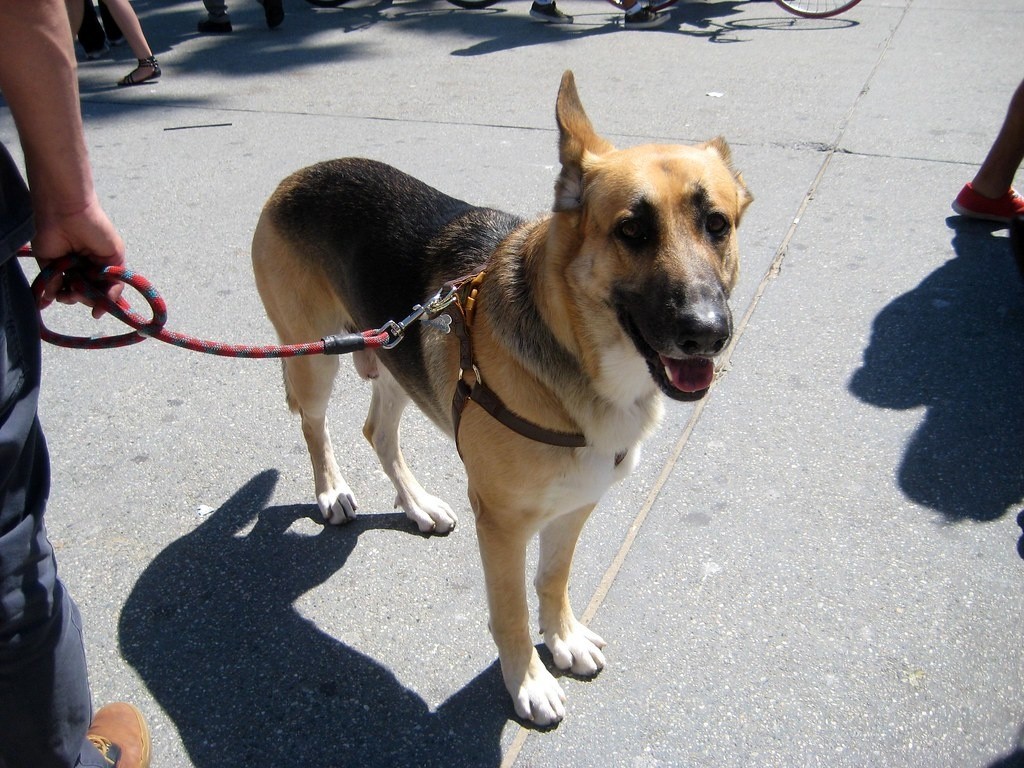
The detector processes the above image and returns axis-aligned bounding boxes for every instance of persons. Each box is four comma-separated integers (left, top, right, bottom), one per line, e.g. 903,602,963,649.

529,0,672,29
0,0,150,768
198,0,284,33
952,80,1024,217
65,0,164,86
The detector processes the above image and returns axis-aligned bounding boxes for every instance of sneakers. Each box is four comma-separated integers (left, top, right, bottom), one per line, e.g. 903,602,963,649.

951,182,1024,222
530,2,573,24
624,7,671,30
87,701,151,768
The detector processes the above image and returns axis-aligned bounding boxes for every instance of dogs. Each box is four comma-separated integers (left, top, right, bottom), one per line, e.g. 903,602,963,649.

249,71,754,730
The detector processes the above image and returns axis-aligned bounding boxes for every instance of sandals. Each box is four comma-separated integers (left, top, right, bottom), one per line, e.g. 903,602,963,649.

118,55,161,86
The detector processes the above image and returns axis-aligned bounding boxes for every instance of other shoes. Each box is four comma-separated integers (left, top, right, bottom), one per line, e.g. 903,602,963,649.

197,19,232,33
264,0,284,29
87,43,110,60
106,35,126,48
